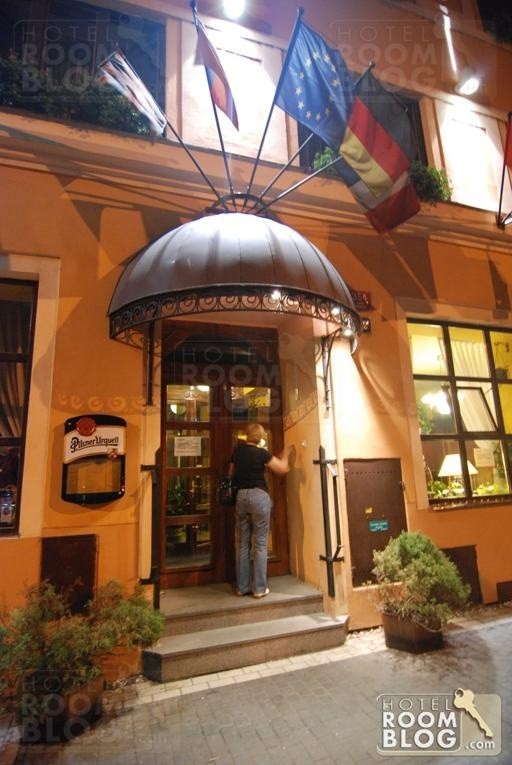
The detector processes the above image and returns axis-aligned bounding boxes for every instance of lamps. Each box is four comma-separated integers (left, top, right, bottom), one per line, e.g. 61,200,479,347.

437,454,479,486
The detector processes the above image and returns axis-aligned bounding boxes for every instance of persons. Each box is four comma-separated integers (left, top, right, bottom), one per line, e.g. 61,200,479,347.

226,422,296,598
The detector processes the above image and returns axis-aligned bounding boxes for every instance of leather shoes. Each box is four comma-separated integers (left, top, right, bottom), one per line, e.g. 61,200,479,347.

254,588,269,598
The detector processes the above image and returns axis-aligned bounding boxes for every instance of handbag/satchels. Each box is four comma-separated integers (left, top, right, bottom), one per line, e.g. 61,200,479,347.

218,480,238,504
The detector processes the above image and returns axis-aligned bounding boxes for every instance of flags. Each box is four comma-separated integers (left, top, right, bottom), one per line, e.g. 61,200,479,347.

97,49,169,148
333,159,422,235
275,18,355,154
332,69,419,197
192,16,239,129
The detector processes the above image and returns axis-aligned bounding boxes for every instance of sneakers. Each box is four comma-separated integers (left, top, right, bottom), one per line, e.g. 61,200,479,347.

236,590,252,595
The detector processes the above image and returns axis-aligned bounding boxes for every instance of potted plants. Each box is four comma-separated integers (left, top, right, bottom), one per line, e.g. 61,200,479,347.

370,529,472,654
0,578,165,745
